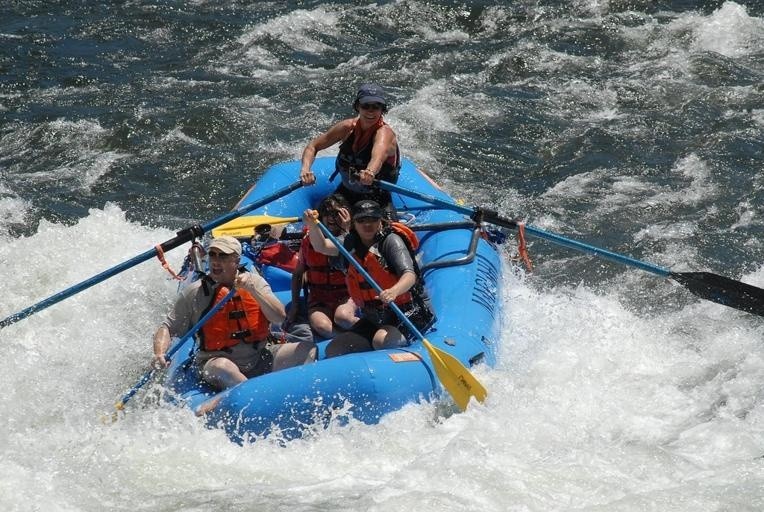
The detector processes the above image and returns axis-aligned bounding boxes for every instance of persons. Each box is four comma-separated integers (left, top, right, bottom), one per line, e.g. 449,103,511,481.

150,235,320,390
283,193,362,339
298,83,401,223
298,198,435,358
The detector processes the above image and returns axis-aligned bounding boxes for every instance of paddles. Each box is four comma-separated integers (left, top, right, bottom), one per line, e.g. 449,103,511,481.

211,200,463,239
312,210,486,411
349,166,763,318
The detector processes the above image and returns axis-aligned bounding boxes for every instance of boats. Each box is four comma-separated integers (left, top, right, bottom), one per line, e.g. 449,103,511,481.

158,153,502,445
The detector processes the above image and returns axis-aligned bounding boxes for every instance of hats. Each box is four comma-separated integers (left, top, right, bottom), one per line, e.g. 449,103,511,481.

352,200,382,220
209,236,242,256
319,194,344,214
357,84,386,104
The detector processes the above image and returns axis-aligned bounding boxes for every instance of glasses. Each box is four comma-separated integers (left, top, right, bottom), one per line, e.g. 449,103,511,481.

360,103,381,109
208,251,229,257
